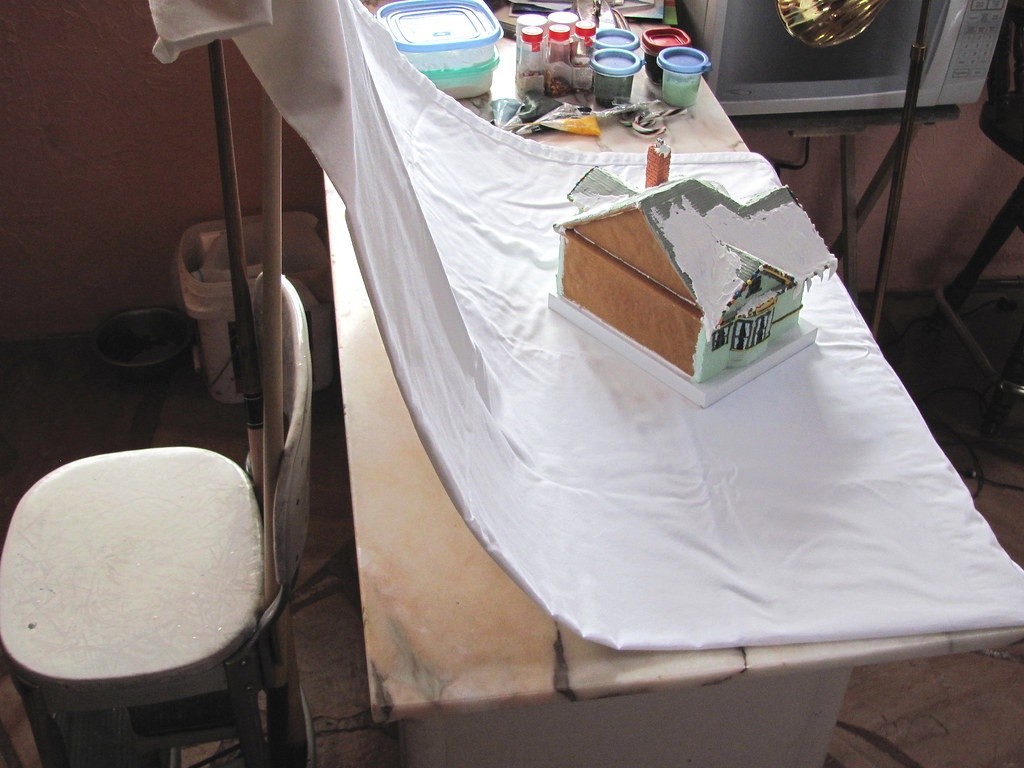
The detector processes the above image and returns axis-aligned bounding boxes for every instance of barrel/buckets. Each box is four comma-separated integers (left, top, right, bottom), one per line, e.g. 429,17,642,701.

177,210,336,404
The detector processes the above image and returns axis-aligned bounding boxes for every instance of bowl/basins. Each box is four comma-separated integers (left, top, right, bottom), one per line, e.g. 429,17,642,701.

90,306,194,379
376,0,504,99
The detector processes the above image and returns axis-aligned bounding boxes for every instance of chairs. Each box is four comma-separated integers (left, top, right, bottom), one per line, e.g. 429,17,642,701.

0,271,312,768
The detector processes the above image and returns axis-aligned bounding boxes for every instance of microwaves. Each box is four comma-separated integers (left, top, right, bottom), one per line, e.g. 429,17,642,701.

678,0,1008,116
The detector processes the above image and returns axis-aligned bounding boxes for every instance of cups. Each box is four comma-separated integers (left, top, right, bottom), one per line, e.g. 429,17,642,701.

589,48,641,109
641,28,692,85
594,28,641,52
656,47,709,107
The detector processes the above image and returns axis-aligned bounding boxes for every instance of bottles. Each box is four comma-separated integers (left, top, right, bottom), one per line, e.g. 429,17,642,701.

188,212,319,281
546,12,579,97
573,20,597,93
514,14,548,97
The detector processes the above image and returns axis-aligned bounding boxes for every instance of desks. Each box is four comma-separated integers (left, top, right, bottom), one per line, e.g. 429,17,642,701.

324,1,1024,768
